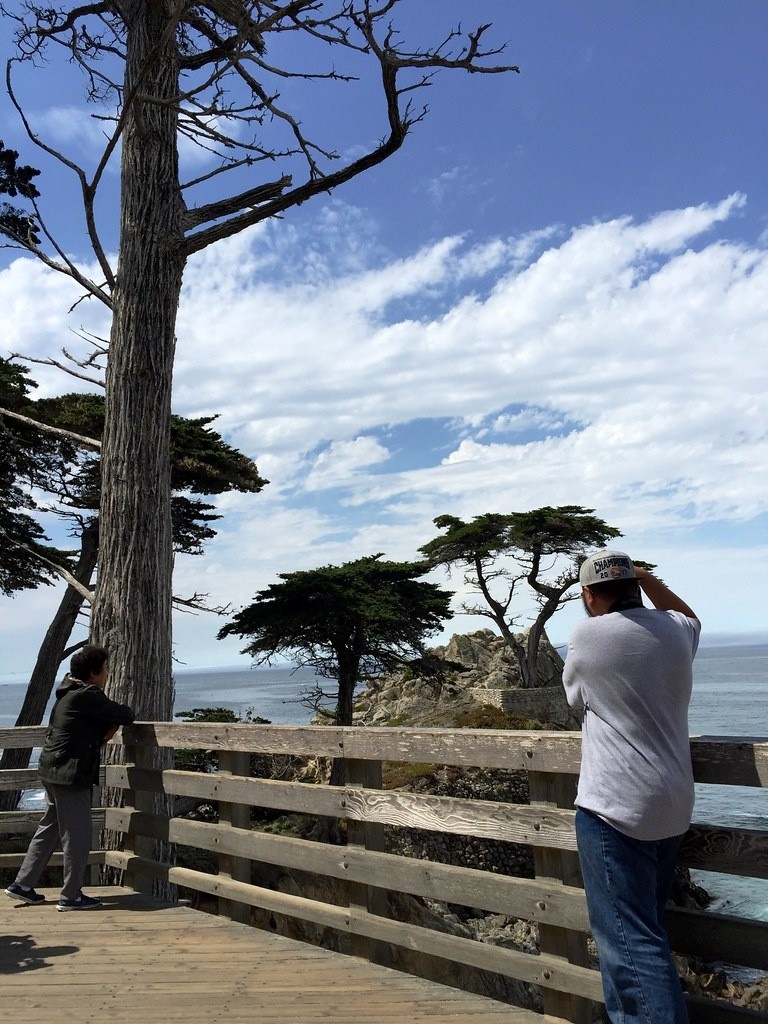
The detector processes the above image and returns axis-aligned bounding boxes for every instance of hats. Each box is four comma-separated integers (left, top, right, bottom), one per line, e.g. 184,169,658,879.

579,549,644,587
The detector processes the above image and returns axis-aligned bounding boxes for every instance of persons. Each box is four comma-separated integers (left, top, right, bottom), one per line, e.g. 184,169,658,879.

560,549,702,1024
4,646,137,913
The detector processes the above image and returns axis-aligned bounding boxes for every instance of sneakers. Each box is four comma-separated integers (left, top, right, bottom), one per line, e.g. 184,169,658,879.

56,894,101,912
4,882,45,905
